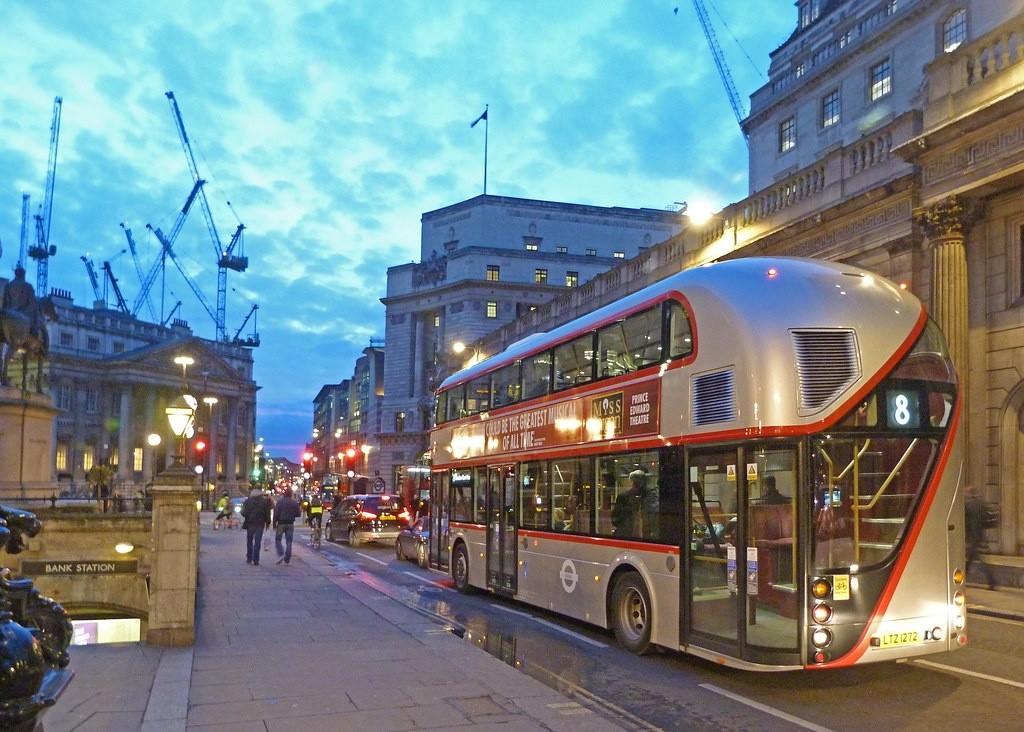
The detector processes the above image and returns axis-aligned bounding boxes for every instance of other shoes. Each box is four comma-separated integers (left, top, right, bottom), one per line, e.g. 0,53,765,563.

247,558,252,562
284,562,291,565
254,560,258,565
276,554,285,564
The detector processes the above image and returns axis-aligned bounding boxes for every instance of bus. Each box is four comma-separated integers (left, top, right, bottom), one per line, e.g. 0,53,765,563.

318,485,336,511
401,467,433,520
428,256,968,672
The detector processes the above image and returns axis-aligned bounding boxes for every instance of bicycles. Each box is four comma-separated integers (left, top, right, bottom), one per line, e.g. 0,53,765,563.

213,514,242,532
306,515,322,550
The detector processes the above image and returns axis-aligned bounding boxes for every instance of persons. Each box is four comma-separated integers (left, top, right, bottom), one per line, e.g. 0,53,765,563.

215,482,327,546
728,476,791,541
273,489,301,566
963,486,999,590
111,490,126,513
409,494,430,523
240,489,271,565
529,365,567,395
3,268,40,338
554,495,578,531
612,470,661,540
332,494,342,511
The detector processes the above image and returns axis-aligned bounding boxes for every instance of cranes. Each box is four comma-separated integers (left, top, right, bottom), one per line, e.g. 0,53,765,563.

14,90,262,348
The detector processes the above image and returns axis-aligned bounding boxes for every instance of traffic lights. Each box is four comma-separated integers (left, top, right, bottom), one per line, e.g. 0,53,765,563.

302,450,313,469
255,468,261,479
196,439,206,460
346,448,356,472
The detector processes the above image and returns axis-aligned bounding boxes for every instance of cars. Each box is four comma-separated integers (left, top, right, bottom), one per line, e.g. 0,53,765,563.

394,515,465,570
325,494,410,549
219,497,248,513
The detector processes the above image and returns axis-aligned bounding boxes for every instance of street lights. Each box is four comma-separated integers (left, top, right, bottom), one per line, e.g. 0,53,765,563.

204,396,218,511
165,395,193,470
147,434,161,481
175,356,194,395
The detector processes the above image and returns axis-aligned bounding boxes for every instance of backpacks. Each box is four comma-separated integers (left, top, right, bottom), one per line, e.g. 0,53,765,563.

310,498,322,507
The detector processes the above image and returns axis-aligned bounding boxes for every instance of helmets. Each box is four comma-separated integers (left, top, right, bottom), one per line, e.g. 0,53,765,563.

222,490,228,498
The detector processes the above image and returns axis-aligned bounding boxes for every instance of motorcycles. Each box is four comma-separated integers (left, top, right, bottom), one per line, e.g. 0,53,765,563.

2,506,75,732
